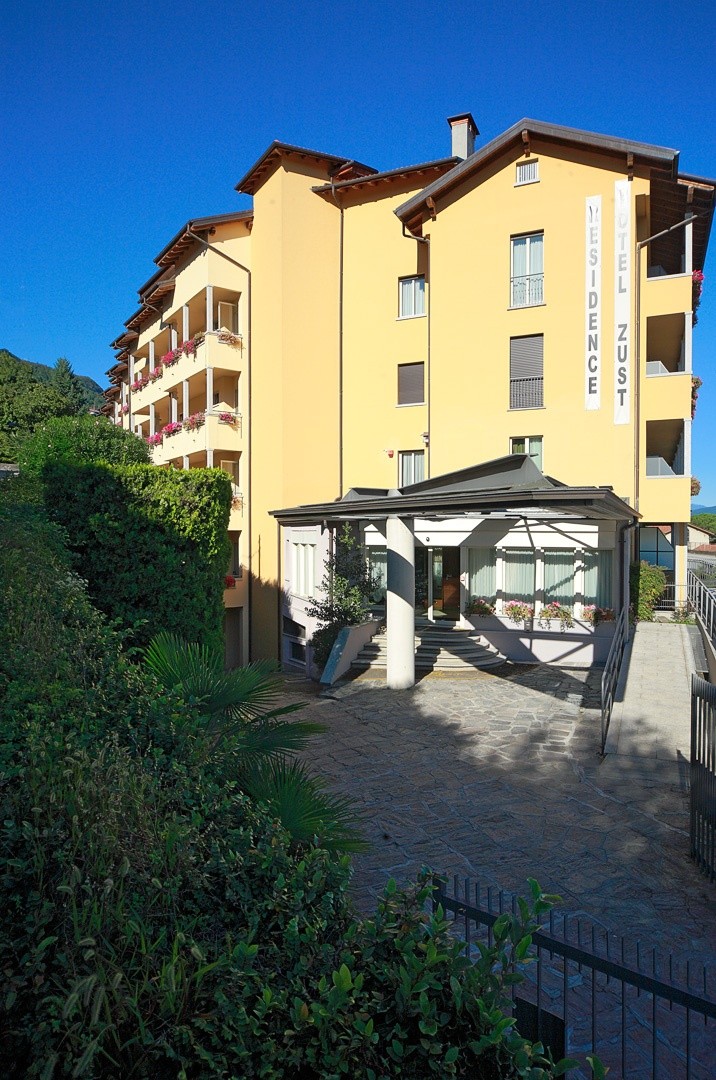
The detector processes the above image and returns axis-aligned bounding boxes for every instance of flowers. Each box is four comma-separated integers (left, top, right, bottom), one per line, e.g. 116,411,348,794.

225,576,236,589
583,604,615,626
540,601,573,628
691,269,704,326
504,600,535,622
691,375,702,417
467,598,494,616
116,328,242,443
